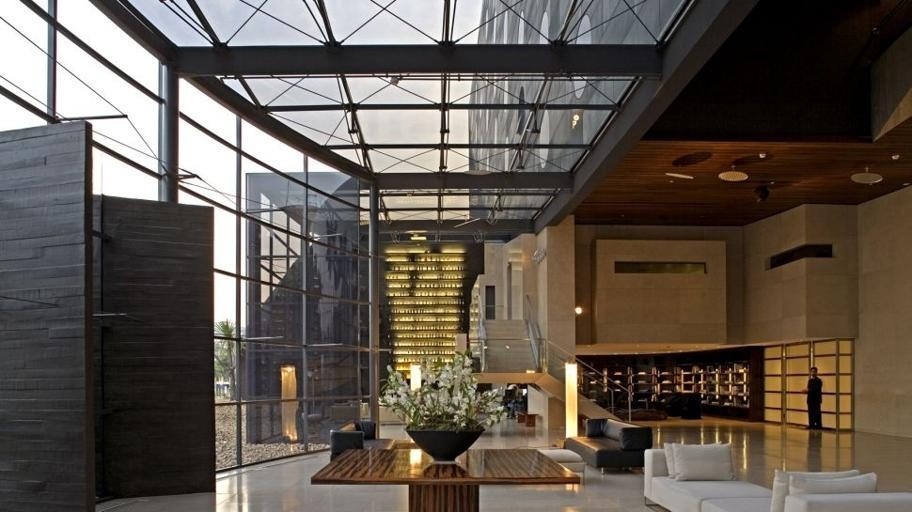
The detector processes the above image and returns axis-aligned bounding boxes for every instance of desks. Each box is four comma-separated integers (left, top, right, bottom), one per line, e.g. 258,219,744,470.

310,447,581,510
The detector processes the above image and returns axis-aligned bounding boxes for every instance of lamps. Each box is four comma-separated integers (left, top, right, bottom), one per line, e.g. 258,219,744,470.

712,161,758,187
564,358,579,442
849,162,890,187
410,362,424,409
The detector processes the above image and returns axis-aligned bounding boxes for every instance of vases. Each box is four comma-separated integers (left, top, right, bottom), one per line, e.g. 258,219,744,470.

406,416,489,462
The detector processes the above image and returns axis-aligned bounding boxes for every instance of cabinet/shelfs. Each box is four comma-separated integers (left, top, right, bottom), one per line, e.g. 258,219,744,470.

583,358,755,421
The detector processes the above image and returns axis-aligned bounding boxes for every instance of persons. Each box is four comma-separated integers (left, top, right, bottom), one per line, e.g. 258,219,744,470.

801,367,823,429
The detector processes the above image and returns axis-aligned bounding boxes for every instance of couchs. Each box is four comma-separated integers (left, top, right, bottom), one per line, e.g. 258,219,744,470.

328,415,390,459
644,448,912,511
563,417,653,476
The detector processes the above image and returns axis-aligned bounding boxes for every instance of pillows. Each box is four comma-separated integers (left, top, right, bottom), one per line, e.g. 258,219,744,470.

769,468,862,511
788,472,878,496
663,440,723,479
672,442,738,481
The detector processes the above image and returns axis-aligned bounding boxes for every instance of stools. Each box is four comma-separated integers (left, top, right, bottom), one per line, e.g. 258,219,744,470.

539,449,586,484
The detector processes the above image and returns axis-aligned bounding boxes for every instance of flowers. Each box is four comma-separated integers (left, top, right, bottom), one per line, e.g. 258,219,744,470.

378,347,529,426
